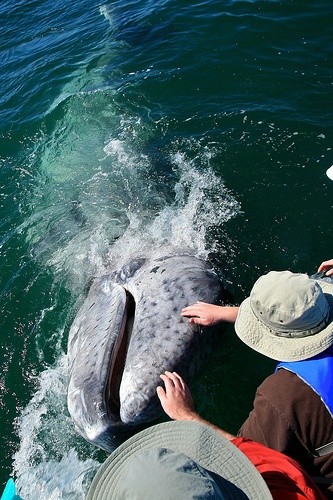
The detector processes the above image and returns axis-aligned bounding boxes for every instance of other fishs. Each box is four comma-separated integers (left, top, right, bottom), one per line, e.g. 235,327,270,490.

67,248,219,454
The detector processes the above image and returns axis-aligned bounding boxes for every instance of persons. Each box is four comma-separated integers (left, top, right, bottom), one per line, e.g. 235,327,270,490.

84,370,328,500
180,270,333,500
317,259,333,276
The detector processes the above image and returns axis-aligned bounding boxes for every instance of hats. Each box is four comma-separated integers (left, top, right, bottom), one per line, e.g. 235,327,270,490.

326,164,333,181
88,417,273,500
234,270,333,362
